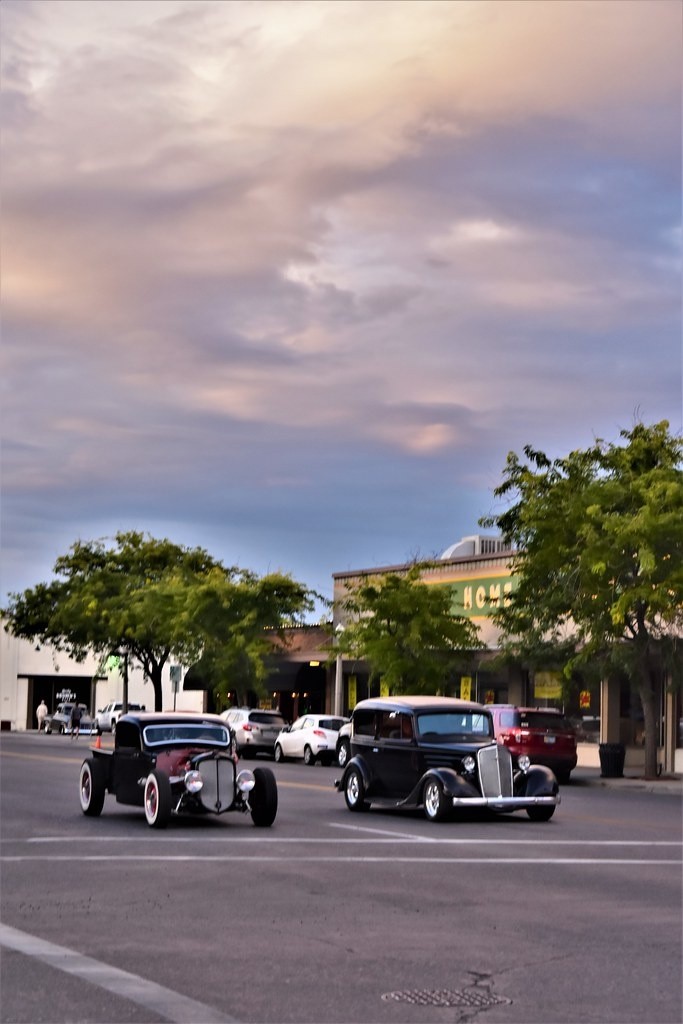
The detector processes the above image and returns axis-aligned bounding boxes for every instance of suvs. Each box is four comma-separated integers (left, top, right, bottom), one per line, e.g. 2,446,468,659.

212,708,287,760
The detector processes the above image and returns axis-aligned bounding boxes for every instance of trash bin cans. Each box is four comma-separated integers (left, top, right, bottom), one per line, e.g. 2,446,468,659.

598,743,626,778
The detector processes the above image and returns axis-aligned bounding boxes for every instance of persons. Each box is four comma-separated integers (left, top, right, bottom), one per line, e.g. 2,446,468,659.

69,699,82,739
36,700,49,735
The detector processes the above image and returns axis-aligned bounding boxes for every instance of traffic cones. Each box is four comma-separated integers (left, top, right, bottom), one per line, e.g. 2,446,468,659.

94,734,103,750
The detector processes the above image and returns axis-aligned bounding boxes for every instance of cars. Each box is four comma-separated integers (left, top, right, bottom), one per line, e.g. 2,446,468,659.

561,714,683,755
95,701,148,736
79,709,279,830
336,717,352,768
473,704,579,785
274,714,351,767
44,702,100,736
335,695,562,824
